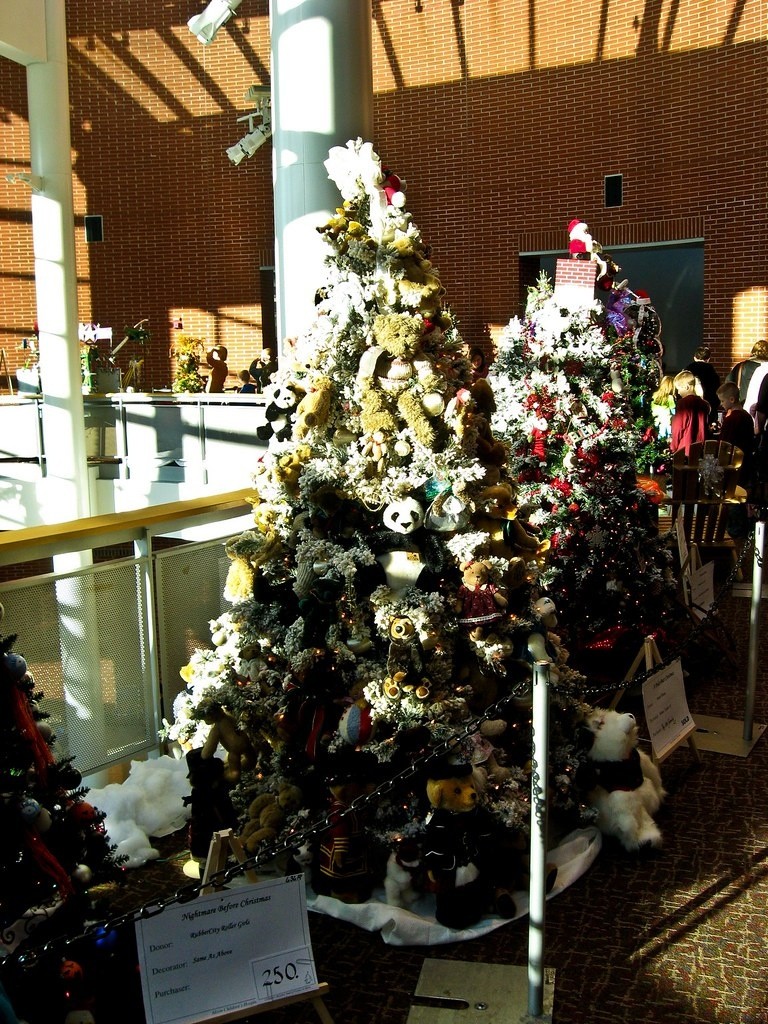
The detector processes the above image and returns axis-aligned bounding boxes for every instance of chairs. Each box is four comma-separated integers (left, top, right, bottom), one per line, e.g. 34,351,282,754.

654,439,748,583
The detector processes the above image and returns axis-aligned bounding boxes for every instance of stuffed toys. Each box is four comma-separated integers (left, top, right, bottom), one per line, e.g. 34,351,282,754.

3,188,649,1022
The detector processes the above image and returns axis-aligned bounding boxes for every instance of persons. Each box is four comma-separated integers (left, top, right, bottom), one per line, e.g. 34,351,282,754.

716,382,755,487
755,373,768,446
648,376,676,443
668,369,711,464
724,339,768,405
682,348,719,422
743,361,768,417
